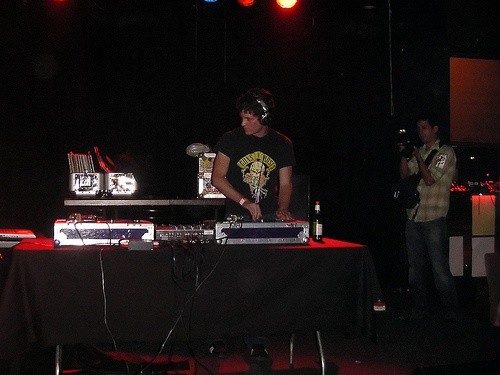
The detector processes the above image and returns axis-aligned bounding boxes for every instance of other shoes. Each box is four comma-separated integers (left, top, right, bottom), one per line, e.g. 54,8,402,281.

398,308,424,320
446,312,458,328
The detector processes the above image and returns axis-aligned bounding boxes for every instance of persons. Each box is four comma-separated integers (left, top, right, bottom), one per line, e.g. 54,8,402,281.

110,139,171,226
213,87,299,375
396,115,461,331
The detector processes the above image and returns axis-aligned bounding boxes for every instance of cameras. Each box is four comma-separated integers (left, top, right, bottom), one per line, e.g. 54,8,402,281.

400,128,415,158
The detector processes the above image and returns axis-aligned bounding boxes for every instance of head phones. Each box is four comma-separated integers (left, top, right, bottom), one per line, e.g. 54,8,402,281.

249,91,273,125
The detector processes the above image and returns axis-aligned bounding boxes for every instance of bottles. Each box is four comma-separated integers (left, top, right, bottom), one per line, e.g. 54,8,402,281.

311,201,323,242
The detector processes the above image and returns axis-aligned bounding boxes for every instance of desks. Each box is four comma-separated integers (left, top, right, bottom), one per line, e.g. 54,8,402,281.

0,228,387,375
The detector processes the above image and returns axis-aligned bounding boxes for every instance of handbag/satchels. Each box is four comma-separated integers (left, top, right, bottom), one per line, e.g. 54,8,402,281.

393,173,422,210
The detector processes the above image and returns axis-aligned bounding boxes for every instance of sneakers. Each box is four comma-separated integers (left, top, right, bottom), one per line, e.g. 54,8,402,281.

248,344,268,361
